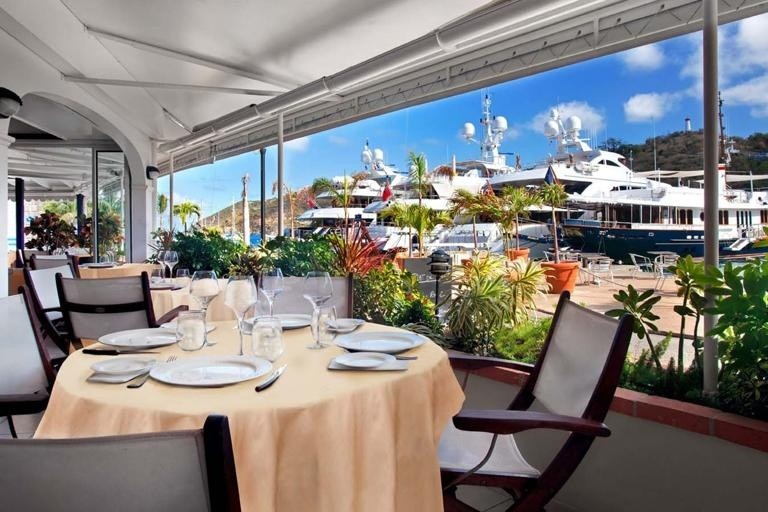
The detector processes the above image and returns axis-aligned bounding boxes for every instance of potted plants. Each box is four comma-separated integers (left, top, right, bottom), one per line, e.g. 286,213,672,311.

382,203,419,270
487,184,542,262
449,190,488,267
538,183,582,294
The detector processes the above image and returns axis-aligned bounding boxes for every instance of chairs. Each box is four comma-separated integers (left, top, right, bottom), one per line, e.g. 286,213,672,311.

0,415,239,509
7,249,195,354
1,284,56,439
437,293,632,509
256,273,353,317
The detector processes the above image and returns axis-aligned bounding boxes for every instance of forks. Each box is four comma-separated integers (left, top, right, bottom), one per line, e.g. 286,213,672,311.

128,355,177,389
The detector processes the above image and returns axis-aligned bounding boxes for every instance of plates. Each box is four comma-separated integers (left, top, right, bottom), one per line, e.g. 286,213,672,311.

150,282,170,290
97,327,186,347
149,354,272,386
162,322,215,333
88,357,159,375
334,331,427,352
325,319,365,327
87,261,115,267
244,313,312,330
335,351,395,369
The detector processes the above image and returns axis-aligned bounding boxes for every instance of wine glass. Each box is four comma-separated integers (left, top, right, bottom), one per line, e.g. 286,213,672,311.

100,239,115,264
260,268,286,315
157,250,178,284
224,275,258,356
190,270,222,345
301,271,333,348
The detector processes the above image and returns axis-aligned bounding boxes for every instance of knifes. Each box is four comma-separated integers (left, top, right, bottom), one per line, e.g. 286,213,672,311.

255,362,288,392
82,348,161,355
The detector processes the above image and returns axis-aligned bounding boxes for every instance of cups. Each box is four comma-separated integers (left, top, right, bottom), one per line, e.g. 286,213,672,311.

311,306,337,346
177,310,208,350
176,269,190,289
252,317,285,361
53,246,86,255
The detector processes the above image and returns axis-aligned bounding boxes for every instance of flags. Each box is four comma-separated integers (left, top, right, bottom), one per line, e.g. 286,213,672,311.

543,166,560,186
382,180,393,202
306,192,315,209
